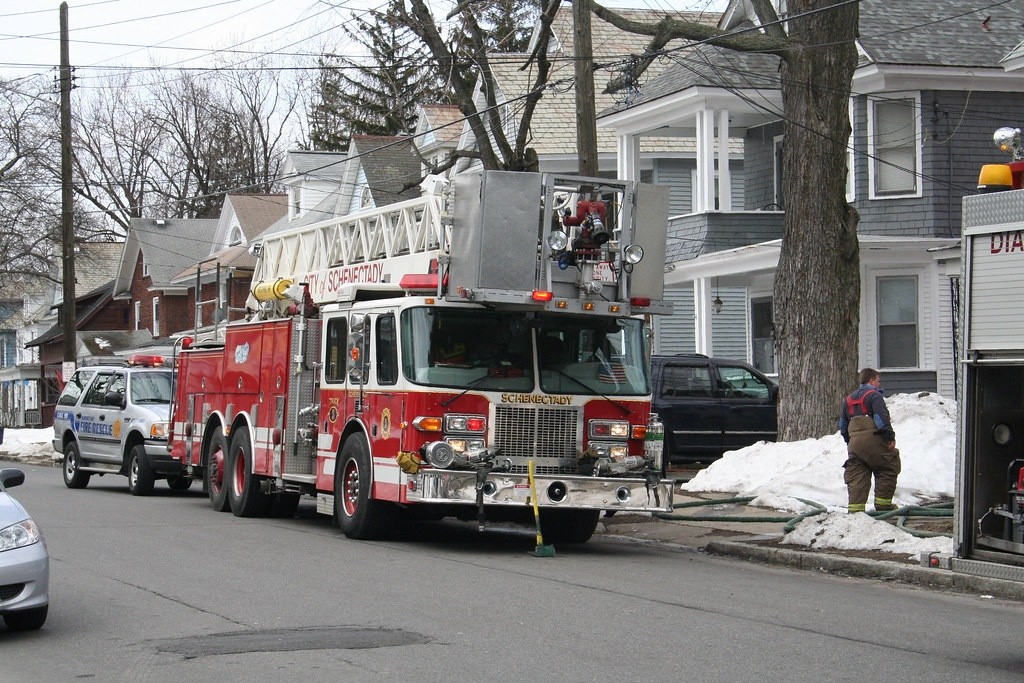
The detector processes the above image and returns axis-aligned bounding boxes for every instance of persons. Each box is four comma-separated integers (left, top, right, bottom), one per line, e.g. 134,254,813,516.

840,368,901,514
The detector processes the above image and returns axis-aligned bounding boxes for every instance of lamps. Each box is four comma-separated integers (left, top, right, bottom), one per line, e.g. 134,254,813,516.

713,277,723,313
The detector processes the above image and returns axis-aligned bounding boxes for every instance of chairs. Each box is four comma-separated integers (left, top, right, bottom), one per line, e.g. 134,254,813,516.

537,336,568,369
689,377,707,397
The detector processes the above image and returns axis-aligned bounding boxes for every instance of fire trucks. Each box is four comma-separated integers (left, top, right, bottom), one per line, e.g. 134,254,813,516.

923,37,1024,583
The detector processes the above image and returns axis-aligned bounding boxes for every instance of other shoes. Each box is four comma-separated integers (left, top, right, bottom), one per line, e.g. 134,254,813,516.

848,511,865,514
876,504,898,510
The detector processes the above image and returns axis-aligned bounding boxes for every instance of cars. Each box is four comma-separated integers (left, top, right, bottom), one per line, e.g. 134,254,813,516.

0,467,49,633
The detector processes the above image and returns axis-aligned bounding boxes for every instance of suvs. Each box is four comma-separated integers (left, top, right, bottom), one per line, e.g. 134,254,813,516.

52,352,189,496
649,353,780,464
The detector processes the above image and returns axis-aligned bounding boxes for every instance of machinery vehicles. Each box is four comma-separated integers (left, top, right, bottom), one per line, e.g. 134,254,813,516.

163,169,674,549
